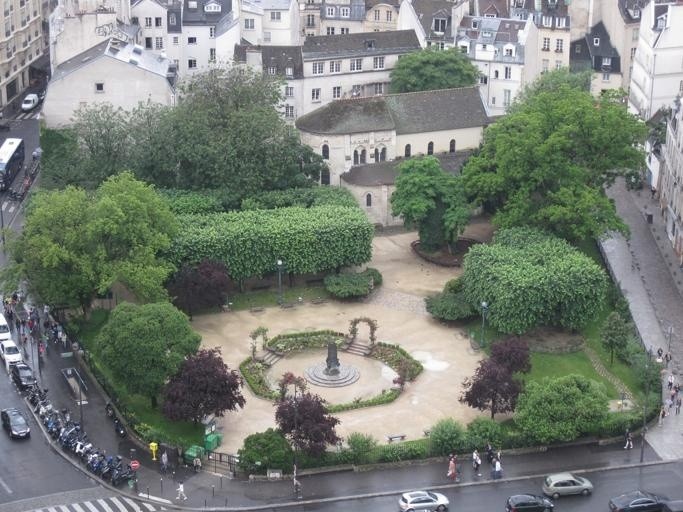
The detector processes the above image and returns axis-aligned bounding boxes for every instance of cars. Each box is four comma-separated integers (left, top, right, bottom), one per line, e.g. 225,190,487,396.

1,407,31,440
542,471,594,499
0,312,37,388
505,494,554,512
397,491,450,512
608,488,683,512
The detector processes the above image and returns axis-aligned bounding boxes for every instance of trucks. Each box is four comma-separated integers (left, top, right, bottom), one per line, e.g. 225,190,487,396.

22,93,39,112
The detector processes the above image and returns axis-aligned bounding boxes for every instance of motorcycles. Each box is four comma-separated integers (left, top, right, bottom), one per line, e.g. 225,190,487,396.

29,387,133,487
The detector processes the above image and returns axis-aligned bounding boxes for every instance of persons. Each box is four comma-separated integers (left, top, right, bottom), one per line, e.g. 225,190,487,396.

160,449,168,474
623,428,634,449
2,290,68,357
446,441,503,482
176,481,187,500
651,187,657,199
655,346,681,426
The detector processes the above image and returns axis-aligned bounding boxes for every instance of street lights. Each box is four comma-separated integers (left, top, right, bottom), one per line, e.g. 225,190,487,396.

478,301,488,348
639,344,666,462
276,255,284,304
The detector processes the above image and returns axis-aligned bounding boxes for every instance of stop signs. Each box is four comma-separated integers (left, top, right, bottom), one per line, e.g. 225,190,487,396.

130,460,140,471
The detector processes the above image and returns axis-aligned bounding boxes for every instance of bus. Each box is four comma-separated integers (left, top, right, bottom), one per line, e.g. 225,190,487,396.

0,138,25,191
